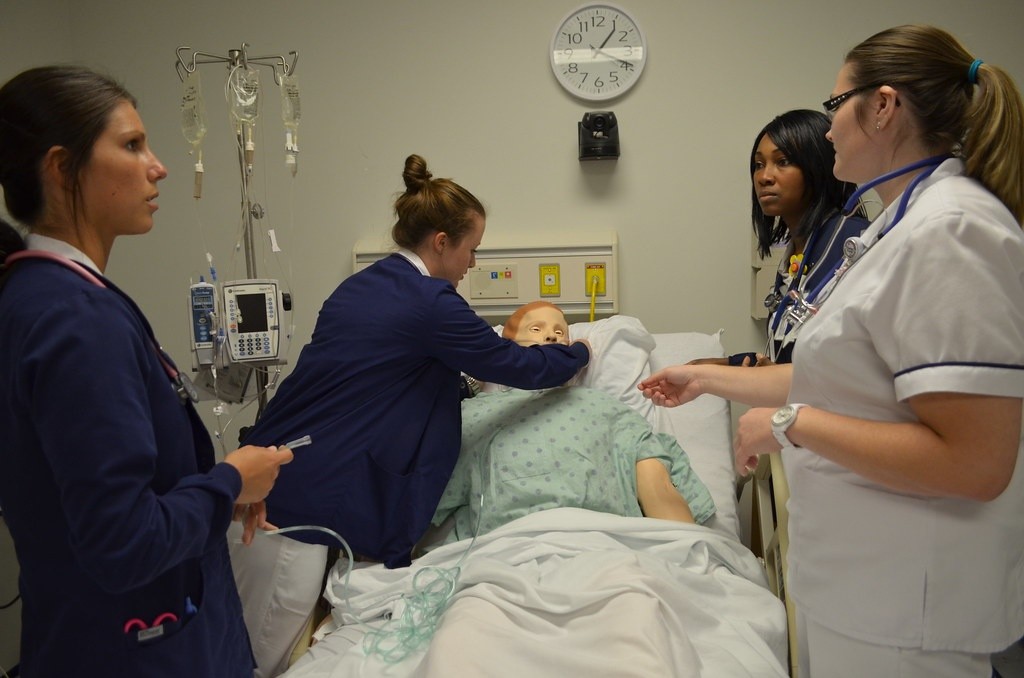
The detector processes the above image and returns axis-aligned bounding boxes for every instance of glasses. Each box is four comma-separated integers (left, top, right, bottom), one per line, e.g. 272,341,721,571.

822,78,900,121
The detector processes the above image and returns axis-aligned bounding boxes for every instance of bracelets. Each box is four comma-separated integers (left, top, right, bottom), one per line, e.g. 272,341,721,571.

464,374,480,395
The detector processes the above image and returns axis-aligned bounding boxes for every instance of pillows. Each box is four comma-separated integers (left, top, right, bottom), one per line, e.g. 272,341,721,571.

480,314,657,432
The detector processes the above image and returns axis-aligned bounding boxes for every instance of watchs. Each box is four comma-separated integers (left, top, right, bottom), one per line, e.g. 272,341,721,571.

771,403,808,447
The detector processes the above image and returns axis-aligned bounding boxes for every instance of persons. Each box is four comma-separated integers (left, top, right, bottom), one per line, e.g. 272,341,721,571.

687,109,872,364
434,303,715,526
0,65,293,678
635,25,1024,677
239,154,593,566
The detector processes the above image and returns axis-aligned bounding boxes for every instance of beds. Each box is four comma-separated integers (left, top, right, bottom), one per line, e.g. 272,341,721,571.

276,335,799,678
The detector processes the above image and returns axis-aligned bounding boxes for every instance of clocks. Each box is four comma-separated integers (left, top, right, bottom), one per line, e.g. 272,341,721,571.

549,5,645,102
